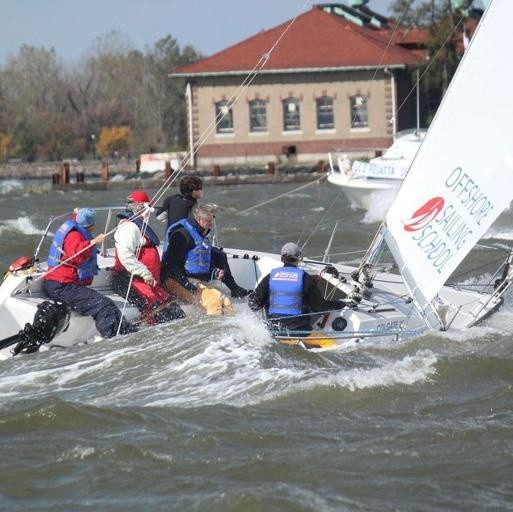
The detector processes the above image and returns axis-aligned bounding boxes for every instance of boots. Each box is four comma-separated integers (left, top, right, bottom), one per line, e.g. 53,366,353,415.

223,277,253,298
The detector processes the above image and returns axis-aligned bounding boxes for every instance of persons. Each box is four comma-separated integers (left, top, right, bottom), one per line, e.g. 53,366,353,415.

158,200,234,319
154,175,254,298
42,206,139,341
246,241,347,339
108,191,187,327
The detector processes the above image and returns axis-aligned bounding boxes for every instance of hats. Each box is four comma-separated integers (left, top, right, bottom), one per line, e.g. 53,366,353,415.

76,208,96,228
128,191,155,204
281,242,300,256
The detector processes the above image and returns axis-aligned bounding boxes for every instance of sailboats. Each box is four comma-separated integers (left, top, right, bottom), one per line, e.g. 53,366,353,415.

0,1,513,372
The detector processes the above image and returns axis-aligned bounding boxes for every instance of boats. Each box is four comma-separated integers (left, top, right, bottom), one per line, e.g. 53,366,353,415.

325,68,513,220
